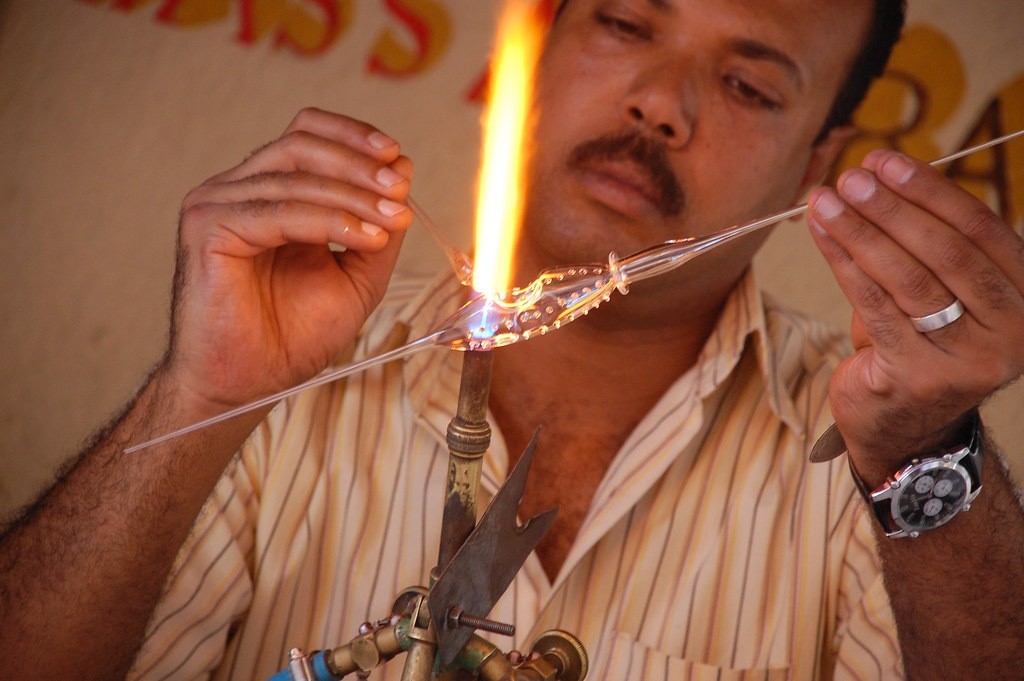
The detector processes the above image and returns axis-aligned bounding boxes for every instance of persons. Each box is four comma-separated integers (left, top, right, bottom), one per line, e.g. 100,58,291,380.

0,0,1024,681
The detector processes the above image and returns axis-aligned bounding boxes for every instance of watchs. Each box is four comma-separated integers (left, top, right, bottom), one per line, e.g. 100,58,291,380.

809,405,986,539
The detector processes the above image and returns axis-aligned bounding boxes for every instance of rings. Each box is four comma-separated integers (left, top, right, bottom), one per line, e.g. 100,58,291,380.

910,298,965,333
